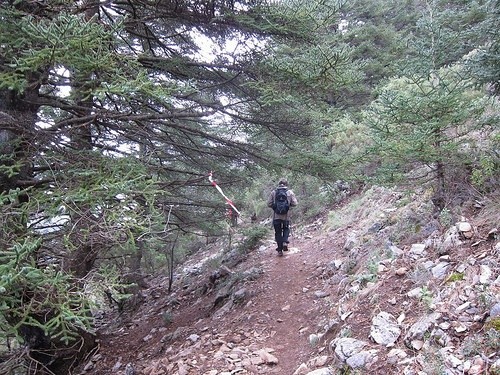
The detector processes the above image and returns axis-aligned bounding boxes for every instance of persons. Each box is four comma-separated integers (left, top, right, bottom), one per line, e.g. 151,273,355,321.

266,178,299,257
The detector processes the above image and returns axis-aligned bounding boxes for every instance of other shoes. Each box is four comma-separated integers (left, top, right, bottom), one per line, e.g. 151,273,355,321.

277,249,283,256
283,242,288,251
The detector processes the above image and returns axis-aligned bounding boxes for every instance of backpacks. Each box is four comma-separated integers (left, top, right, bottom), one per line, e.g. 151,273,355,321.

272,188,291,215
